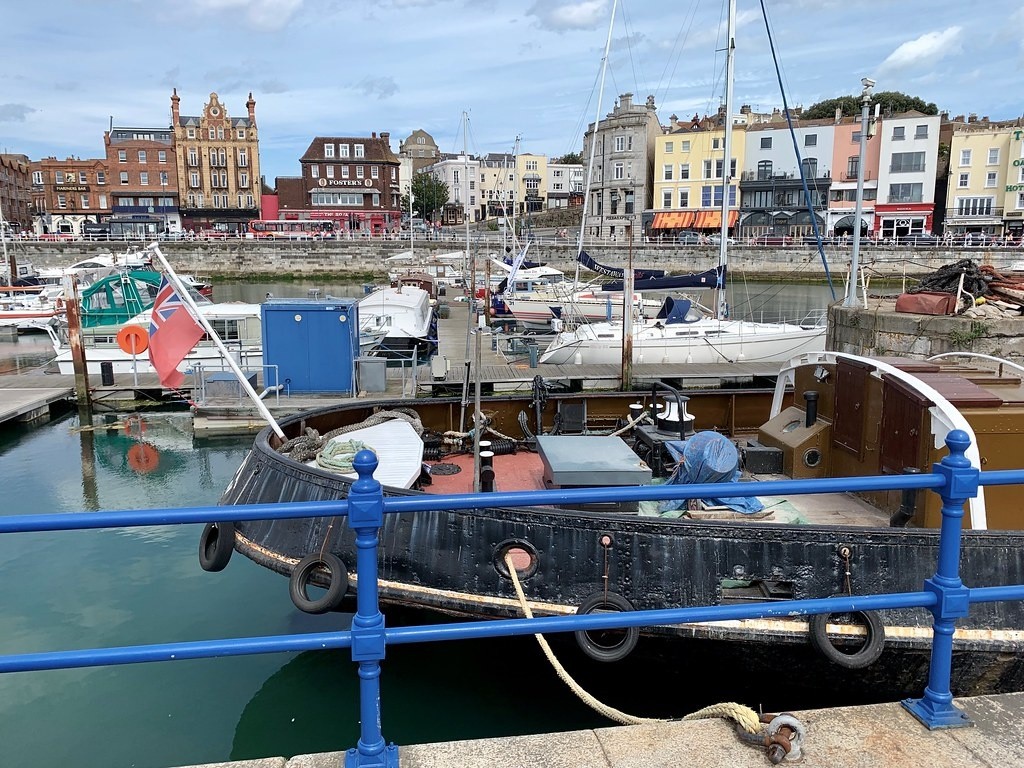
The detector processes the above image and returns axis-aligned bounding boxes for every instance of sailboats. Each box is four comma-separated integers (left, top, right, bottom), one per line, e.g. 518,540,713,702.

529,0,829,387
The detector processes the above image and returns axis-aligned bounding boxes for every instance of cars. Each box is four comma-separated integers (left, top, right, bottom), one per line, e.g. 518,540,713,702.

749,232,797,241
195,229,231,240
799,234,833,245
900,233,939,246
158,230,187,241
39,232,81,239
834,236,876,247
950,231,1016,246
705,234,740,242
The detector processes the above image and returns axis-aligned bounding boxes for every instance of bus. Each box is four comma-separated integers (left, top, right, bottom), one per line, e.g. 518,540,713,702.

248,220,340,239
0,221,22,241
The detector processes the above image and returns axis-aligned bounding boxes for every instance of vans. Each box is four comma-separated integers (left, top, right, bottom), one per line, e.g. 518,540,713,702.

82,223,111,242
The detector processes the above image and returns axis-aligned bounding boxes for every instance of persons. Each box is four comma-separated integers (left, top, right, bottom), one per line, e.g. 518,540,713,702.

841,230,847,245
554,226,567,238
753,231,757,246
697,232,707,244
944,231,1016,246
9,228,37,242
379,228,389,236
181,227,194,241
57,228,63,241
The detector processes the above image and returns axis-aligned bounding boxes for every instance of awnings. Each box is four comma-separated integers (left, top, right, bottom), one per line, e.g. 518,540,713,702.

651,211,739,228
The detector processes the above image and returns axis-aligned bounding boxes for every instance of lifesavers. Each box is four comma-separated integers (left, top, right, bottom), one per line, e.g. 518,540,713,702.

199,522,232,571
576,592,639,662
290,553,348,614
812,593,885,668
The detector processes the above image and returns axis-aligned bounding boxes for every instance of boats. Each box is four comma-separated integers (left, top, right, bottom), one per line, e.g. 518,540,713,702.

350,1,674,366
197,326,1023,696
0,216,263,372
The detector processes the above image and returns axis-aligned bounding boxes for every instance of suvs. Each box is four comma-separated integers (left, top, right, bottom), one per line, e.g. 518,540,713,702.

678,231,710,244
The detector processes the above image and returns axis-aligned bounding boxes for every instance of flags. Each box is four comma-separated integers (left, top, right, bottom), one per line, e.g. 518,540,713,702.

147,270,207,390
